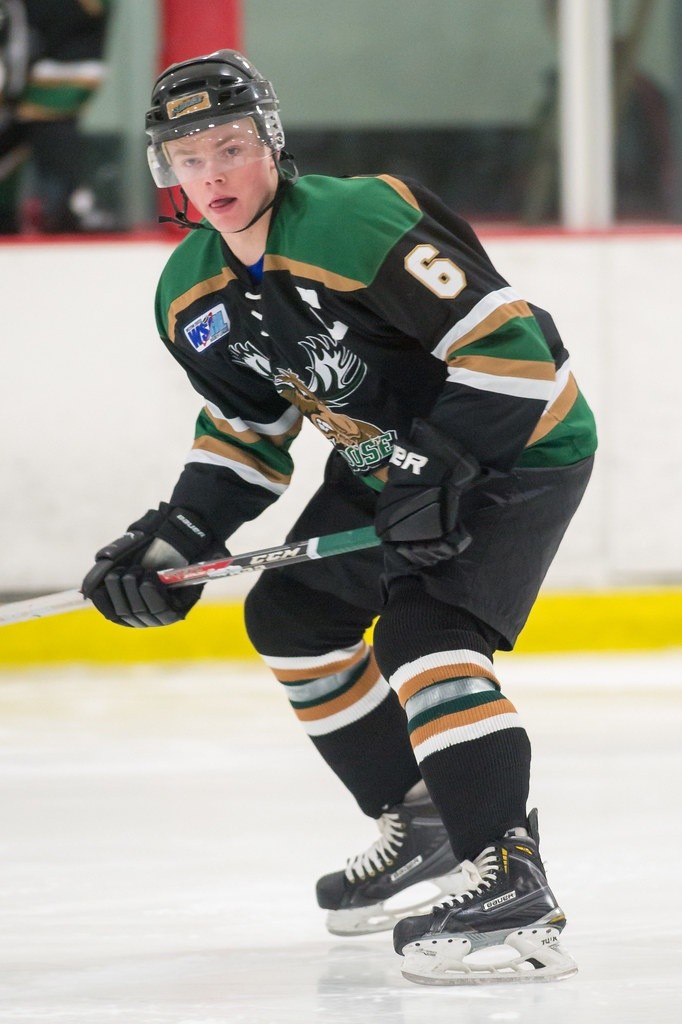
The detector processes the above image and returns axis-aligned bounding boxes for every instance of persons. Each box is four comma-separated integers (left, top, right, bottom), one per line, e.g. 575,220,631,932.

79,49,598,985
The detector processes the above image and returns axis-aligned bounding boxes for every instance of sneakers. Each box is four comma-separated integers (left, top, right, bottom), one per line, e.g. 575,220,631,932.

316,795,462,937
393,808,578,984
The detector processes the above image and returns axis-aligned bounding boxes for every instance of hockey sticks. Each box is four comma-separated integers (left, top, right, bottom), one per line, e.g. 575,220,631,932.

0,517,384,627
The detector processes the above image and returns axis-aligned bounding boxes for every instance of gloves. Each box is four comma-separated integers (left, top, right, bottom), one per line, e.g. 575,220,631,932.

82,501,232,627
375,416,476,571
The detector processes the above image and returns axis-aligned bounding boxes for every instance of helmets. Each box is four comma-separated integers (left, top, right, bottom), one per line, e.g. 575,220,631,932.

144,48,285,188
